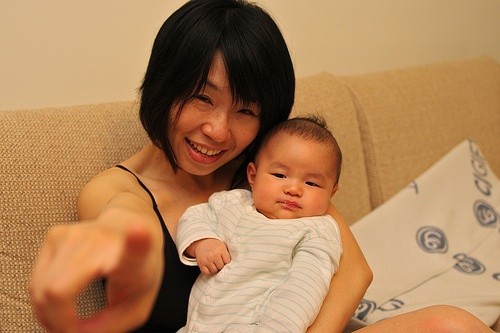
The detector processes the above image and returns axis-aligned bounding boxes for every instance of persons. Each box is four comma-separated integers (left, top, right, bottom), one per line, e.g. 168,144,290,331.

28,0,498,333
173,112,343,333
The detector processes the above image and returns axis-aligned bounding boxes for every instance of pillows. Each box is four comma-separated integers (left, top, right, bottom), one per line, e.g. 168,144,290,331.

349,138,500,328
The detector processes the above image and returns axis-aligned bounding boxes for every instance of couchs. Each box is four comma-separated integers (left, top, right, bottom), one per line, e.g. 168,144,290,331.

0,55,500,333
